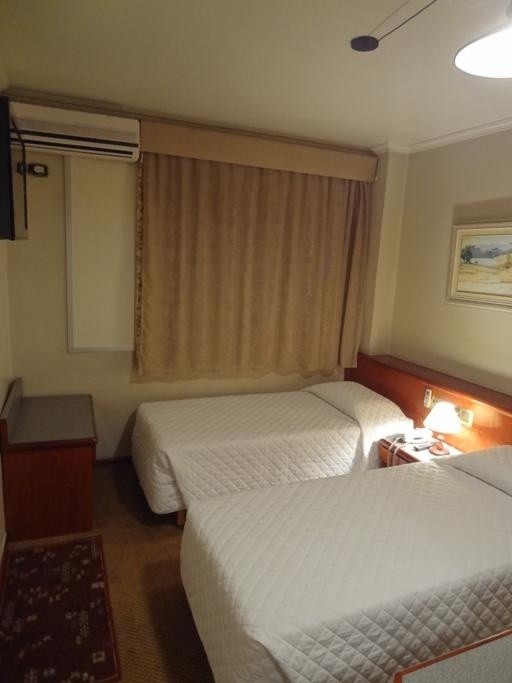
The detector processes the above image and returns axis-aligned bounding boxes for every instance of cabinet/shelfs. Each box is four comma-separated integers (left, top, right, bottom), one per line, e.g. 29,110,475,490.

5,376,99,544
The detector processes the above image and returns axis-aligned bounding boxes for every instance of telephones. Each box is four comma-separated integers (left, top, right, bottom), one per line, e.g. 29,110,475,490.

404,428,433,444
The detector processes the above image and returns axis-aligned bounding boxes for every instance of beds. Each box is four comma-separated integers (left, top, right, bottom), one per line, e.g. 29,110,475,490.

131,390,414,526
179,462,511,681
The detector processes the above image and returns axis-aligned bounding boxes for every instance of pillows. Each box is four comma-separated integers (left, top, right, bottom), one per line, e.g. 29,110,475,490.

303,379,405,428
450,445,512,497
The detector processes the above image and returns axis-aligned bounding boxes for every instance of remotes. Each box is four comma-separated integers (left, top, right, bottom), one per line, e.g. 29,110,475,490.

414,441,434,450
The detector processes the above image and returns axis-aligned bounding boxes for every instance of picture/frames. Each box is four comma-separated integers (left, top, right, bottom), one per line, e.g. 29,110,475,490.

445,220,512,313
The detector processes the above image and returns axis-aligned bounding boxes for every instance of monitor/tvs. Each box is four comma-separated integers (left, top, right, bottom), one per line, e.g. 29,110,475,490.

1,96,30,241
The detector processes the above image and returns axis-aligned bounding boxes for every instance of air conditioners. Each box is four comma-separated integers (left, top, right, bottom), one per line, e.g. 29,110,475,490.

7,100,142,164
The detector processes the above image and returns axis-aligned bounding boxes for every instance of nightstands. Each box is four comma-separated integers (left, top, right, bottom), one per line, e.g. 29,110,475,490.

380,432,462,467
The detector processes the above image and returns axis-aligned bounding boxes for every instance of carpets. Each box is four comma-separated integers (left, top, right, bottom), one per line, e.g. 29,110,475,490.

1,534,123,683
